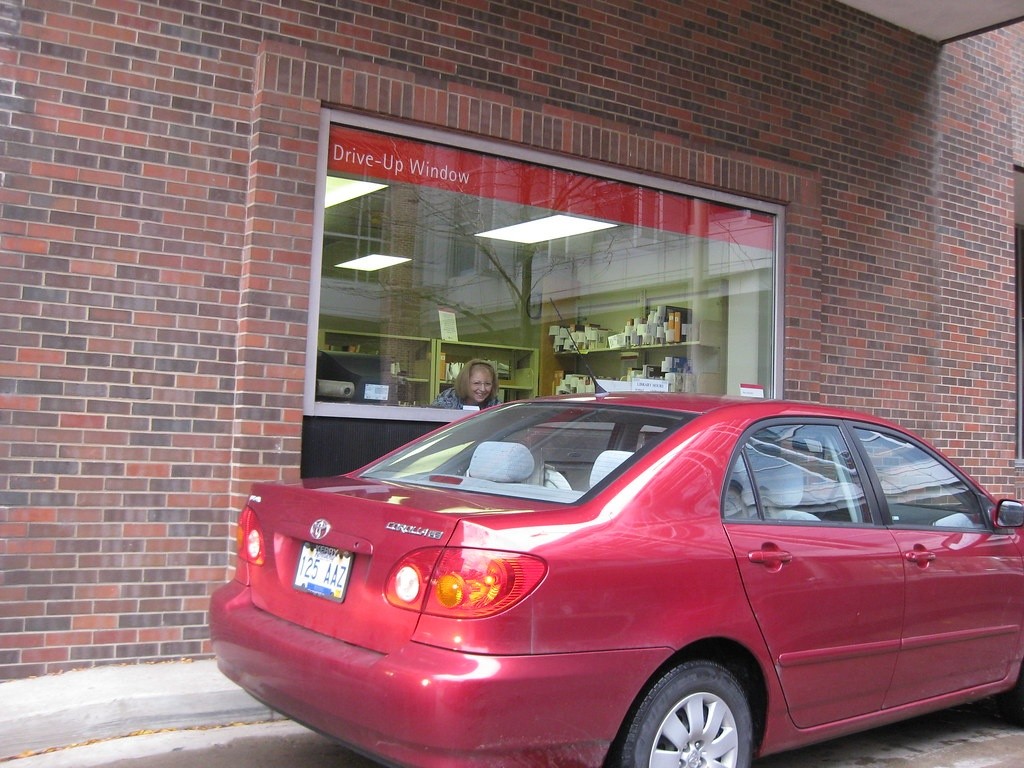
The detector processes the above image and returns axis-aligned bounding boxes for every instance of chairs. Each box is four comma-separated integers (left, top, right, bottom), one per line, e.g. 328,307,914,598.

745,457,822,521
468,440,635,522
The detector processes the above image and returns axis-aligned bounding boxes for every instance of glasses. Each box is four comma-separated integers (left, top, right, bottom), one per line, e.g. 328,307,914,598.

471,381,492,388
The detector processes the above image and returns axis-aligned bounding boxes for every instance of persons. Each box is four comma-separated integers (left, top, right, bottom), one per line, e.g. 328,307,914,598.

431,359,498,409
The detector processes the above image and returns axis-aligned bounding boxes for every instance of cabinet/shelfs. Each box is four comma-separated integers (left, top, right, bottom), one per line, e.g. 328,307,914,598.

318,328,540,409
554,278,729,397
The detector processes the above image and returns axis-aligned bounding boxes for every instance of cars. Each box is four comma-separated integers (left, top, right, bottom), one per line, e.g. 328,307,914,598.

208,392,1023,768
